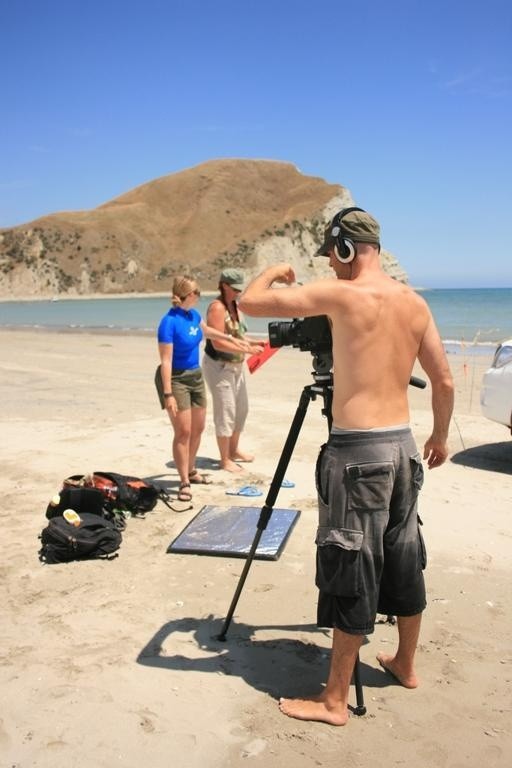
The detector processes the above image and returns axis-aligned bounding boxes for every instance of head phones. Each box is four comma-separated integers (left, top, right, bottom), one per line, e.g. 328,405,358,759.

329,205,363,267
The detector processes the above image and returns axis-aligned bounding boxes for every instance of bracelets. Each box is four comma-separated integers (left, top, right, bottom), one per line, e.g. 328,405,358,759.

163,393,173,398
256,341,265,347
228,334,232,341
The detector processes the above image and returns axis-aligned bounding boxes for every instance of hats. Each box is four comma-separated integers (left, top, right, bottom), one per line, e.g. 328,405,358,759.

220,268,249,292
313,210,380,257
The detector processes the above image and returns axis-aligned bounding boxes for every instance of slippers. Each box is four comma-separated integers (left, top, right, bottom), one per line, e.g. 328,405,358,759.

225,485,263,496
281,478,295,488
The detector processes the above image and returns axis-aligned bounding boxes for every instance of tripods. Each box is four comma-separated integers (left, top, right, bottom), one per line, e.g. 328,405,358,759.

211,382,399,720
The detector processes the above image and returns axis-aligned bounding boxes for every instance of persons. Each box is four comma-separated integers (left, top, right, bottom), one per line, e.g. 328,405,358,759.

156,274,253,502
238,208,454,727
202,268,268,472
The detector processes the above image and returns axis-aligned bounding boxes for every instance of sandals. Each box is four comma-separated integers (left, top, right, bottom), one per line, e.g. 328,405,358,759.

178,483,192,501
189,471,213,484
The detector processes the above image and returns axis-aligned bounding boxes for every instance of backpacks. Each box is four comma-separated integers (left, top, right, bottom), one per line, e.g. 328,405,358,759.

38,472,157,564
